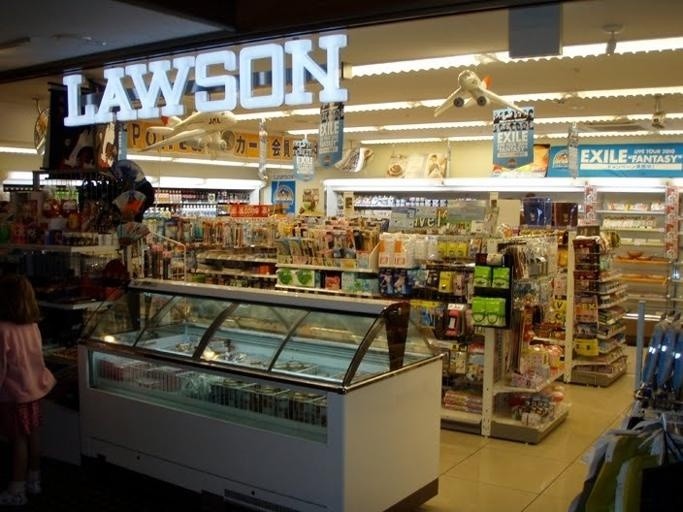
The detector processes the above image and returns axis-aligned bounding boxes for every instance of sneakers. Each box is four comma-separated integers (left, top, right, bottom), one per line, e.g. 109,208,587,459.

26,481,42,493
1,491,27,506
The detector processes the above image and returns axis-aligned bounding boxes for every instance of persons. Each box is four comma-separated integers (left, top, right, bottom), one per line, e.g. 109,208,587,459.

0,274,58,506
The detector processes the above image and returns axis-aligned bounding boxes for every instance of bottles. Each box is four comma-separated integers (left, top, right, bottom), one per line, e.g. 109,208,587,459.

143,189,217,217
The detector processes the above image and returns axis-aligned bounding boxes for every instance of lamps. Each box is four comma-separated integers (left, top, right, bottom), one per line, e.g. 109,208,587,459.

604,38,619,58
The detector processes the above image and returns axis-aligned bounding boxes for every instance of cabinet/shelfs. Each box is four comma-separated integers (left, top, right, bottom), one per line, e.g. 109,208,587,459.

490,362,572,445
336,181,479,224
595,186,683,345
572,232,629,386
142,189,254,220
0,241,187,393
194,206,491,439
